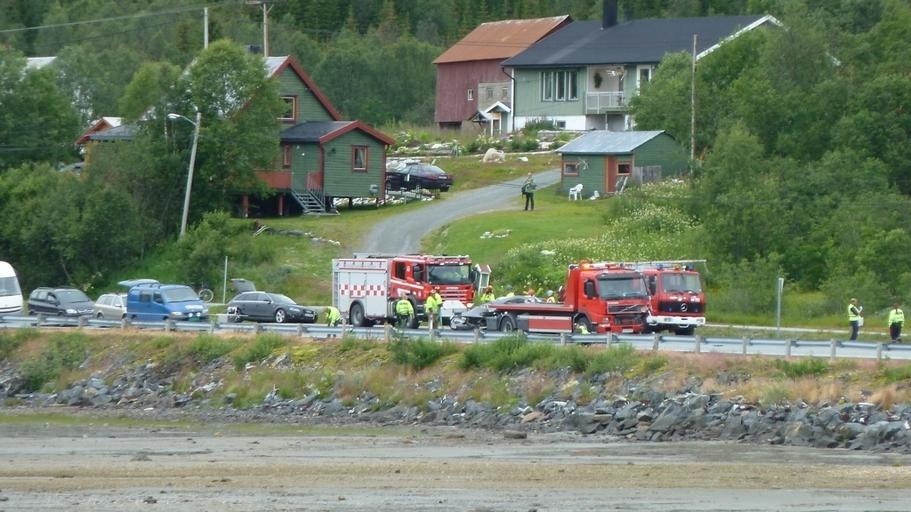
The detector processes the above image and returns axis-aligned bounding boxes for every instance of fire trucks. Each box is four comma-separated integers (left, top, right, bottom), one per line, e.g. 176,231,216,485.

568,259,708,336
483,265,650,337
329,251,480,330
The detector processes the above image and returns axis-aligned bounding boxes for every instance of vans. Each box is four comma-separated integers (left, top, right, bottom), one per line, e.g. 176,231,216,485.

0,260,23,321
117,277,209,323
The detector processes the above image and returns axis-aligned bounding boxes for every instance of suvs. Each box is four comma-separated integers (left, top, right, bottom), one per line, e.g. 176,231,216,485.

27,287,94,326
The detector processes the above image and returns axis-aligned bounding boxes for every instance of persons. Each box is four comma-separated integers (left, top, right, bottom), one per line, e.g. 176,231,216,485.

527,289,536,297
505,285,515,297
848,298,863,341
324,306,342,337
522,173,534,211
425,290,438,329
480,285,496,302
544,290,556,303
396,295,414,330
434,286,443,329
888,302,904,344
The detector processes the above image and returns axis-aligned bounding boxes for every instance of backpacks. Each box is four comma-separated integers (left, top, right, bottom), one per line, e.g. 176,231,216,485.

521,182,528,195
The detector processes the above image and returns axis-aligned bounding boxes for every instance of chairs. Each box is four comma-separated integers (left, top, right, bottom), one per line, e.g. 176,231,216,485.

569,183,584,202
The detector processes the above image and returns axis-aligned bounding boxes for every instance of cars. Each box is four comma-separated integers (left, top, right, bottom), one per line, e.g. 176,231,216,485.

227,289,318,324
94,293,127,325
384,163,453,194
450,294,551,330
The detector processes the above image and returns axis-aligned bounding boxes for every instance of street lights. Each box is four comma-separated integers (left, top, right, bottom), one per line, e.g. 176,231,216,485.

167,108,202,246
